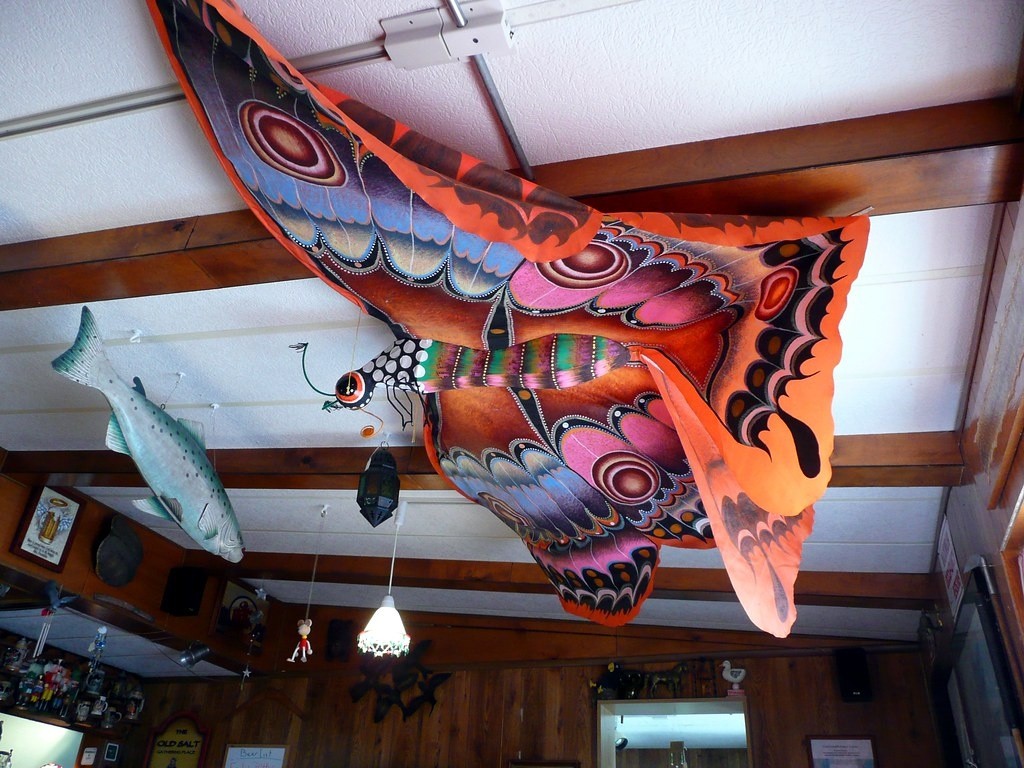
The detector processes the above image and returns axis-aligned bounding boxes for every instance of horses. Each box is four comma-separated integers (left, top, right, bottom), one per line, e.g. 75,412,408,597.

646,662,689,698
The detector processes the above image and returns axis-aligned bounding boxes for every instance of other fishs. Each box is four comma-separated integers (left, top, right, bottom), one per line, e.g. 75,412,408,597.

50,306,245,561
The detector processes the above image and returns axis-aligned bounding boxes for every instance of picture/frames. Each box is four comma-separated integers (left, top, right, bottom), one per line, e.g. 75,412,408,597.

9,486,86,574
507,759,582,768
805,735,878,768
206,575,273,656
221,742,291,768
142,709,212,768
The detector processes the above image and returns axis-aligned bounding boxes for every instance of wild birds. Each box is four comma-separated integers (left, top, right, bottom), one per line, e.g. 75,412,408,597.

352,642,451,723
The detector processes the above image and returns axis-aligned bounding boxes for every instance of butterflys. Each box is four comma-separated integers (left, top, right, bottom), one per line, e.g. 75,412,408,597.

146,1,870,638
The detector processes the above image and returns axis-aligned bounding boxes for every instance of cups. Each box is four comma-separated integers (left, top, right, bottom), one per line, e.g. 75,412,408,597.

38,511,60,545
76,700,91,722
125,691,145,720
91,696,121,729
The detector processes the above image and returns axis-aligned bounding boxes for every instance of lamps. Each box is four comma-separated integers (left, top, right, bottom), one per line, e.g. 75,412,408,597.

356,501,411,658
356,441,401,528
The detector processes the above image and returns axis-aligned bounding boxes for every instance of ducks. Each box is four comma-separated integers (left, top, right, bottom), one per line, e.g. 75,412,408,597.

718,660,746,682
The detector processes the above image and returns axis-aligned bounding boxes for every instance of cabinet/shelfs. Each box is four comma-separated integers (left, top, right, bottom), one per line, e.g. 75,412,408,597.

0,666,144,741
